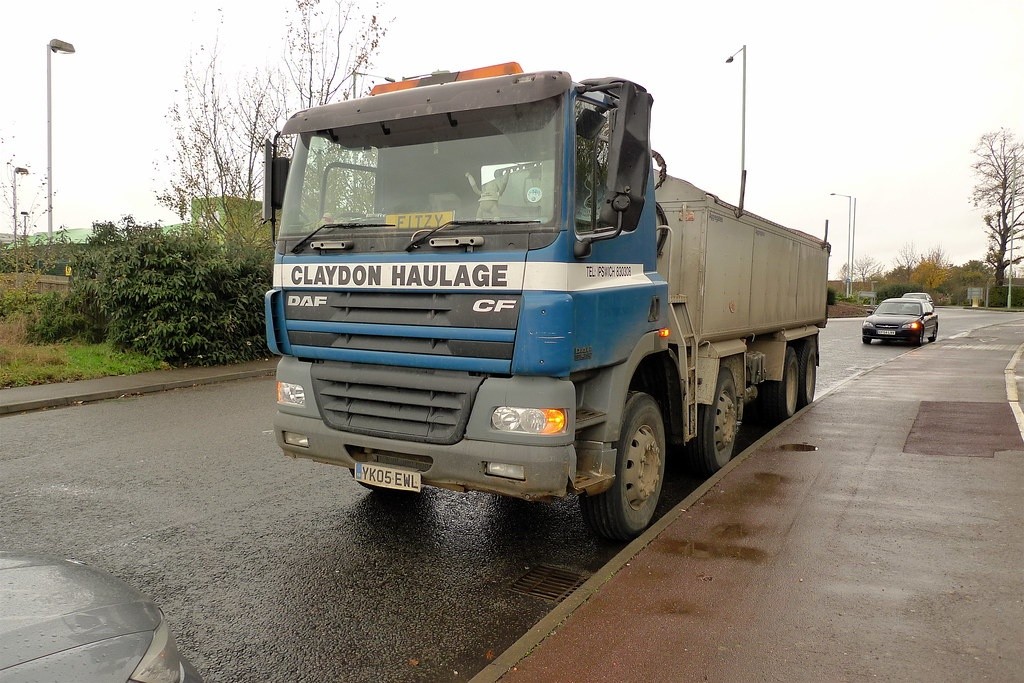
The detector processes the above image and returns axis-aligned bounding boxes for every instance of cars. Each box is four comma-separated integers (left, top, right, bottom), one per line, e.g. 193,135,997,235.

861,297,939,346
902,293,935,311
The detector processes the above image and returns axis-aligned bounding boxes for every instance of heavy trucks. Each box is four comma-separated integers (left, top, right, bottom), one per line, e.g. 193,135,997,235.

262,62,830,544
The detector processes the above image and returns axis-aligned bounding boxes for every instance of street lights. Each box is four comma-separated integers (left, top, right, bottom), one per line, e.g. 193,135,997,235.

14,167,29,280
727,45,747,207
830,193,851,300
21,212,28,268
354,71,395,97
47,39,76,242
